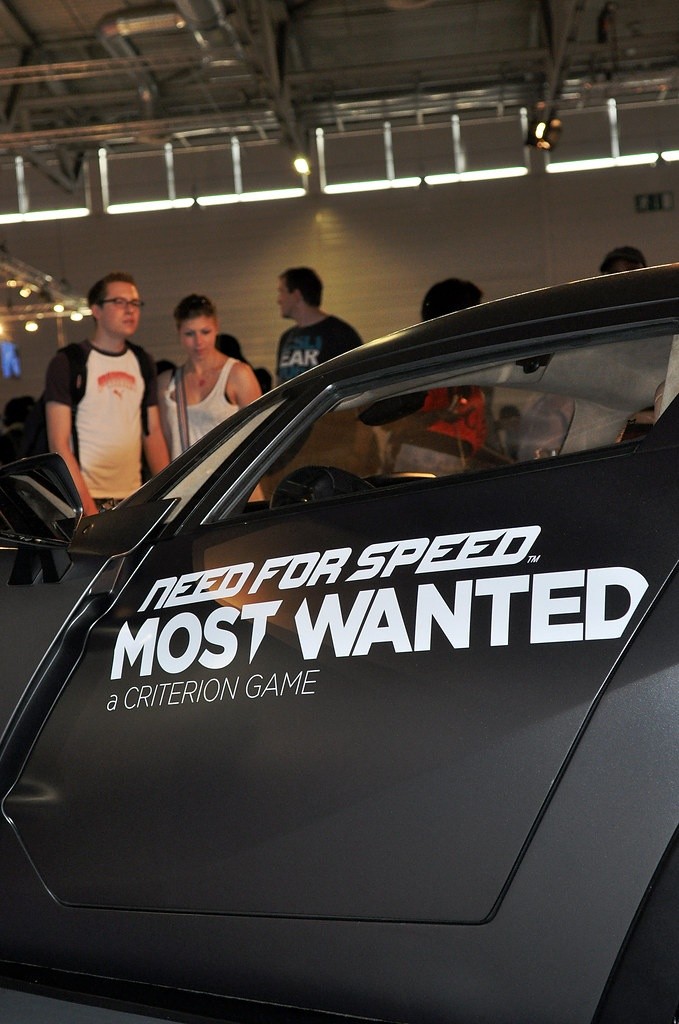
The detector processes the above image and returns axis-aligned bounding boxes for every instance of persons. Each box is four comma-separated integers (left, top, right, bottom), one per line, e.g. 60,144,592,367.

0,395,34,465
368,276,520,477
272,267,375,488
493,245,675,464
156,292,264,503
156,332,274,393
43,271,172,518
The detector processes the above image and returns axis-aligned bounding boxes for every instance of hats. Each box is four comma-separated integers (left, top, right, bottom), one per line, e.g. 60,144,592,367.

601,247,645,273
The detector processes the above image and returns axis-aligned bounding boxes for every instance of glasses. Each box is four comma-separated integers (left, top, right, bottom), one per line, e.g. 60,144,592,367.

91,298,144,312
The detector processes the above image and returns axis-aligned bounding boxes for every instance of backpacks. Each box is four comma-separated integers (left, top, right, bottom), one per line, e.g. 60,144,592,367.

19,340,150,472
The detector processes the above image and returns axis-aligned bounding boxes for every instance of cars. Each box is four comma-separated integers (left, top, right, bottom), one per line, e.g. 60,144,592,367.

0,256,678,1024
